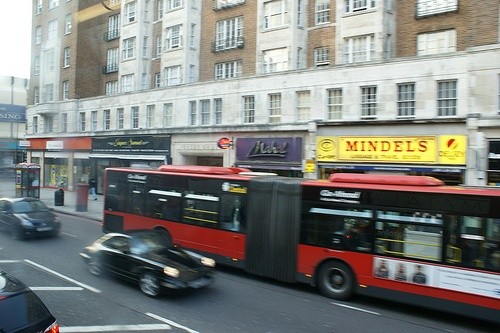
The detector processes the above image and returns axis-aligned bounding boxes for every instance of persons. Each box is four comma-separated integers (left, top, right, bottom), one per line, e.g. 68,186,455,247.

88,176,98,201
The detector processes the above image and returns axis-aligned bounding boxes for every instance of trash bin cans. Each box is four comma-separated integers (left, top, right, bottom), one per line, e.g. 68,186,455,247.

54,189,64,206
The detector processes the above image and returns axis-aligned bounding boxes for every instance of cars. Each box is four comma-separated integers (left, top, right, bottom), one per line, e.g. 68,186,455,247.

0,197,61,241
78,232,216,300
0,269,61,333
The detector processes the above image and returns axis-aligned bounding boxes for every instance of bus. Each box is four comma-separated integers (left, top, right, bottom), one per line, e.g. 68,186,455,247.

102,165,500,324
